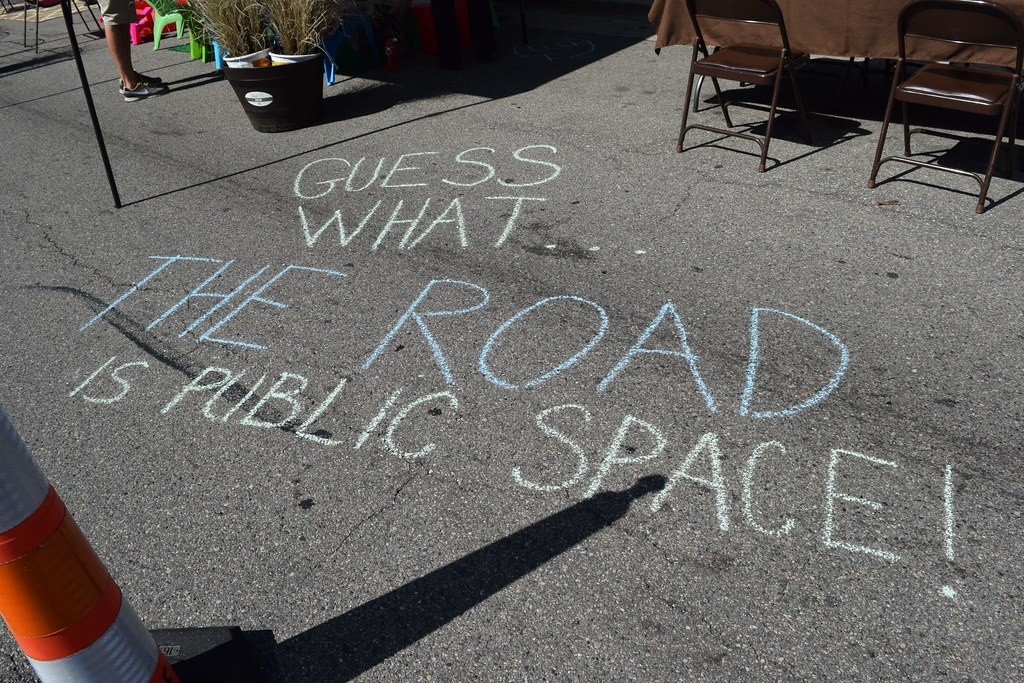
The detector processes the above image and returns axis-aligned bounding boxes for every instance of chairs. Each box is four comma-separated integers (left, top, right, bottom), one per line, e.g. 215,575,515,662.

867,0,1023,214
146,0,190,51
24,0,106,55
675,1,811,172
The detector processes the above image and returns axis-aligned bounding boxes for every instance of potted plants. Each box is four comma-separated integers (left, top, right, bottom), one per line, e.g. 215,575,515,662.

183,0,359,134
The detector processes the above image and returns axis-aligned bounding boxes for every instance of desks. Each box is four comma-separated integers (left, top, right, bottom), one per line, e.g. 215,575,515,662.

654,1,1023,88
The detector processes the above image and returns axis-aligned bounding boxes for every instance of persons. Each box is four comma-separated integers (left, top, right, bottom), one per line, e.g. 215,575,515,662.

95,0,168,102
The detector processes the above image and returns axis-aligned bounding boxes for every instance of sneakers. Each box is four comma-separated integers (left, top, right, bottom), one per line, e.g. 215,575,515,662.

118,73,162,94
124,78,169,102
